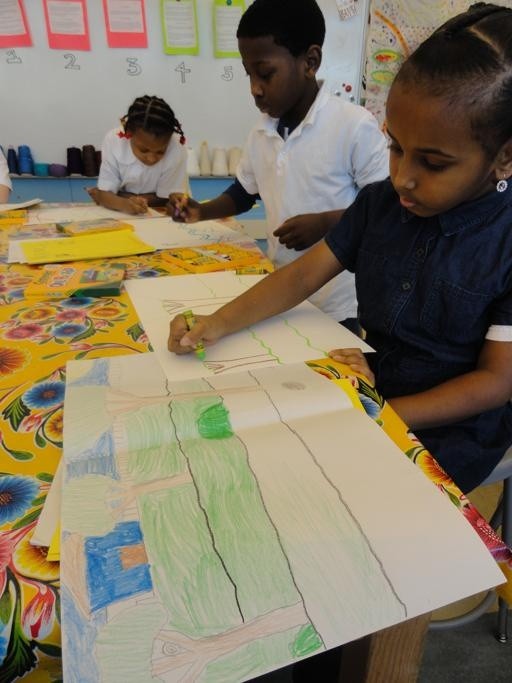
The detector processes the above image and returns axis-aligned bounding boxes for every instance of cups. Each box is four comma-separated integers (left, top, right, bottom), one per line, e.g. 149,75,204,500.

33,161,49,177
49,163,67,177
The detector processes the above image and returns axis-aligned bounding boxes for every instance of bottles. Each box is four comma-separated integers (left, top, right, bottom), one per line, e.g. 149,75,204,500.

16,146,34,174
81,145,98,177
7,148,18,174
66,147,83,173
187,139,243,177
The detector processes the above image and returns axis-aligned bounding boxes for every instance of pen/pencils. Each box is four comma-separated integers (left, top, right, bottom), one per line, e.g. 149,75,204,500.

173,194,188,217
182,309,209,360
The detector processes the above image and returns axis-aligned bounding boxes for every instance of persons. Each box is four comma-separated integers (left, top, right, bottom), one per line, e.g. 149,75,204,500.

167,1,511,497
0,146,14,204
81,94,190,216
161,0,391,339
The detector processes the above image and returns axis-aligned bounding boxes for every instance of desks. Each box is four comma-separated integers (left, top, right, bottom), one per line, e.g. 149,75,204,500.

0,205,512,681
